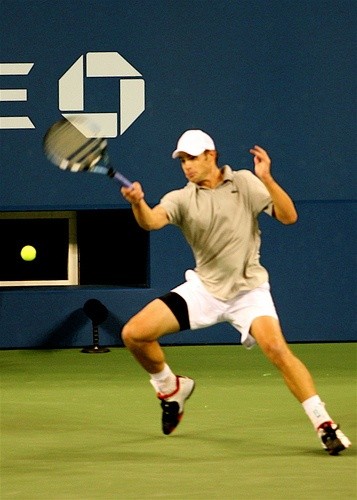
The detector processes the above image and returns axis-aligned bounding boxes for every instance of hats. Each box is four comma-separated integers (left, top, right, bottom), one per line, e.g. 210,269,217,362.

172,129,215,159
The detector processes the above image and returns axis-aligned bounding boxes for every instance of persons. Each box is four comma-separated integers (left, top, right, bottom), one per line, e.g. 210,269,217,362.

119,128,353,454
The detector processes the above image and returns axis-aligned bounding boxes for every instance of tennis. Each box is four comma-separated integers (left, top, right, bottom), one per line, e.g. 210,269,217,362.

20,244,37,262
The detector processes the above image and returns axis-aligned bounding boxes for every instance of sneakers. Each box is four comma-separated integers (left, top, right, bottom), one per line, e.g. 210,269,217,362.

317,421,352,455
158,375,195,435
